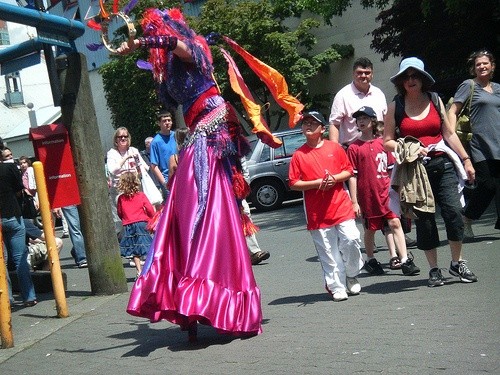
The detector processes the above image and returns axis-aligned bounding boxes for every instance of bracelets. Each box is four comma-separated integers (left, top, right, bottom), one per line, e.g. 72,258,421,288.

461,156,470,162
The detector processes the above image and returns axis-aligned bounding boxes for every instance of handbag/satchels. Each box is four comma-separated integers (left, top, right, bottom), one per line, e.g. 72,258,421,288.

136,159,164,205
446,79,474,149
423,154,445,178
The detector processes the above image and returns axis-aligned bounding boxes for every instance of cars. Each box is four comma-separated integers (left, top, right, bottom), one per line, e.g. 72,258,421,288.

242,125,337,213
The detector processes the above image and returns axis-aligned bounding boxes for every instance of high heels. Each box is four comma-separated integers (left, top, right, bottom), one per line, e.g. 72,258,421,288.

186,316,197,341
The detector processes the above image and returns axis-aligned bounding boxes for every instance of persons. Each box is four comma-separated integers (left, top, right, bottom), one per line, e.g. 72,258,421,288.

117,8,264,335
139,136,165,200
166,127,192,191
347,105,420,277
288,111,364,301
106,126,150,267
241,155,270,265
0,136,37,312
149,111,177,201
382,54,479,287
116,172,157,280
0,147,69,246
444,49,500,239
328,56,417,252
53,204,89,268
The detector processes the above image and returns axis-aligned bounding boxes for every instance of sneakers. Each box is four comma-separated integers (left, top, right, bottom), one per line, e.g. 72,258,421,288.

449,259,478,283
401,251,420,276
428,268,445,287
364,258,385,276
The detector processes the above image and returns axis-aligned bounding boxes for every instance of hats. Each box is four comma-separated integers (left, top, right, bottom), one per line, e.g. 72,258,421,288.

300,110,326,126
352,105,377,118
390,57,435,89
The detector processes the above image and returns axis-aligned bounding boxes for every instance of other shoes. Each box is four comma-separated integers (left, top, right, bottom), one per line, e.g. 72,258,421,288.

332,290,348,301
346,276,361,294
78,263,88,267
372,242,378,253
24,300,37,307
251,251,270,265
405,234,415,247
390,257,402,269
460,213,474,239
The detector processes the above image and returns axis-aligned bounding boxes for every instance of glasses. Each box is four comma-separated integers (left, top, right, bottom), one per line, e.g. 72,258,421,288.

402,73,423,80
116,135,129,138
